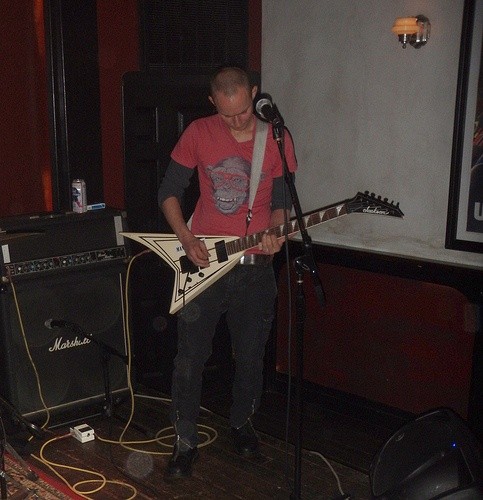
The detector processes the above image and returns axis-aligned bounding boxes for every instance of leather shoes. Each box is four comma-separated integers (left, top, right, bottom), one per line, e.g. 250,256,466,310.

165,443,199,480
228,423,259,457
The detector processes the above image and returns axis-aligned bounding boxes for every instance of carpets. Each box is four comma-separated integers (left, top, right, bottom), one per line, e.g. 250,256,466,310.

0,451,88,500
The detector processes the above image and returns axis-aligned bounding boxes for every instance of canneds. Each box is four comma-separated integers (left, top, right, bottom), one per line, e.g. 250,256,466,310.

72,179,87,213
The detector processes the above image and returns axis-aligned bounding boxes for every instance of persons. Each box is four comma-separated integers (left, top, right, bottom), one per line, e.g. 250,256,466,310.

156,65,300,483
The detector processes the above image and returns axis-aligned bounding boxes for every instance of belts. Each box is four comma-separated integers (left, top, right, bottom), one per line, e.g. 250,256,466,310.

237,254,272,265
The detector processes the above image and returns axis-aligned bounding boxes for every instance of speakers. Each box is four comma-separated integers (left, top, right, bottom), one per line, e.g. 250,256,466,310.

369,406,483,500
0,264,137,427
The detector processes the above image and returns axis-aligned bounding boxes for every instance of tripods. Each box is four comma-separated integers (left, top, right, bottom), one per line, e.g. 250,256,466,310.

70,321,149,438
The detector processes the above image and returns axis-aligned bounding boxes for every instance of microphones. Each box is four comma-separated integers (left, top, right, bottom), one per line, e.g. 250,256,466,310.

256,99,284,128
43,317,71,329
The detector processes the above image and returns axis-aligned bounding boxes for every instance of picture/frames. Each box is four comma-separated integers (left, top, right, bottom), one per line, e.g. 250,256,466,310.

444,0,483,253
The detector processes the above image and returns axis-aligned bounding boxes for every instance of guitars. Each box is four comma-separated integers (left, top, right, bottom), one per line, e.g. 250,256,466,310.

117,190,406,316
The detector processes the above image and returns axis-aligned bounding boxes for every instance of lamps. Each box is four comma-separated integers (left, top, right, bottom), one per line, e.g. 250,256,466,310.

392,14,430,49
